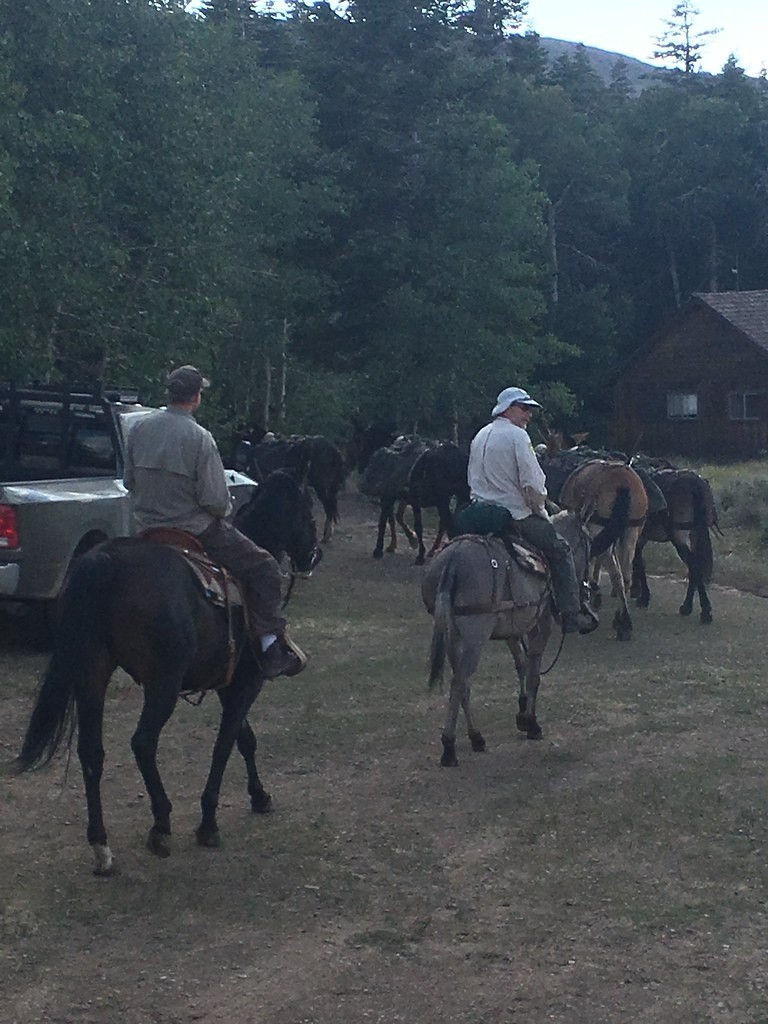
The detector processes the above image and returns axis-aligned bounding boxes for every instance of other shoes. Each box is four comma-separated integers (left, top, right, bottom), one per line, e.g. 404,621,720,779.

253,630,293,675
561,612,592,632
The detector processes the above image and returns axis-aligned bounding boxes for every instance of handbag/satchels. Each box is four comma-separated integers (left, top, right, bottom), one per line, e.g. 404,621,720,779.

448,503,512,537
357,447,407,495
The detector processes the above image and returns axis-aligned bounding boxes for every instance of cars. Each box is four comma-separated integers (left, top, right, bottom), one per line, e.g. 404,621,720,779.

0,381,263,638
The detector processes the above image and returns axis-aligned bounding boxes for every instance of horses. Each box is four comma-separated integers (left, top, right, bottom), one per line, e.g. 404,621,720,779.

5,433,719,878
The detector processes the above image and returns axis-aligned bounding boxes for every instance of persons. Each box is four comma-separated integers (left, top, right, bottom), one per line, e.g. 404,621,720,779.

468,387,593,634
123,365,298,677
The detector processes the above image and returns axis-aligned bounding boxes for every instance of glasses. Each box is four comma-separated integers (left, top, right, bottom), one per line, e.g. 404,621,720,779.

512,403,530,412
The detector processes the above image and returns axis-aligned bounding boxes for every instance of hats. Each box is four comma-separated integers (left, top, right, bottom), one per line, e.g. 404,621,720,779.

491,387,543,416
166,364,210,395
